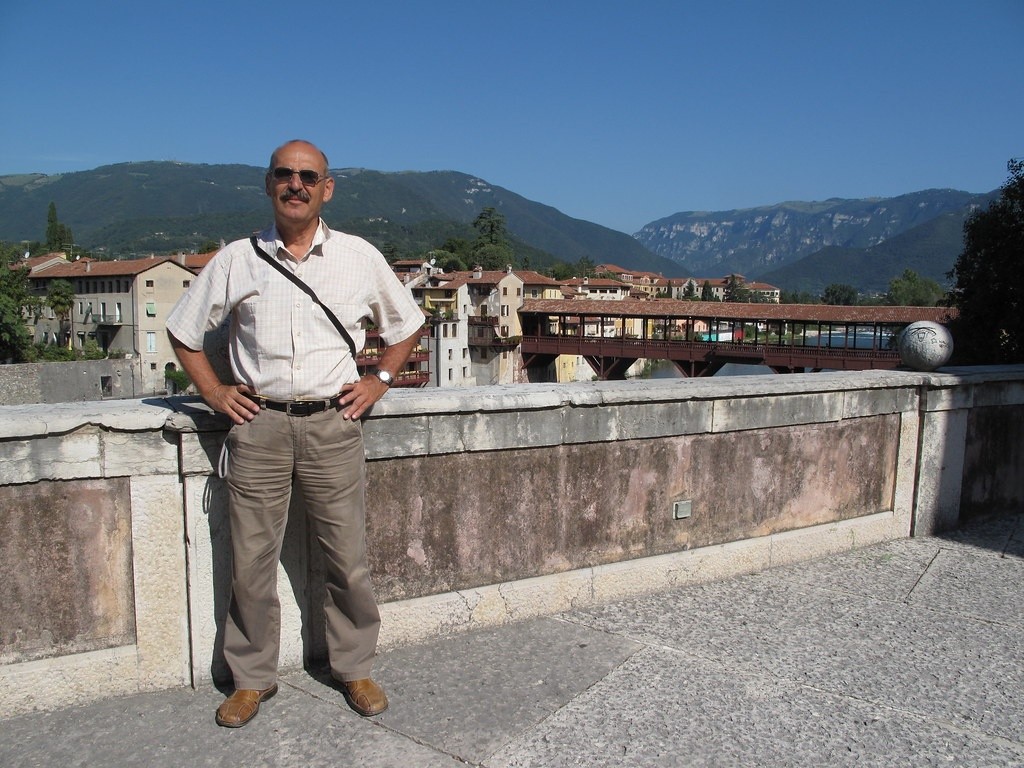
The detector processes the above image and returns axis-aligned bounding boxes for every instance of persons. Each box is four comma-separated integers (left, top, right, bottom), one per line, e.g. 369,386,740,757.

166,140,426,727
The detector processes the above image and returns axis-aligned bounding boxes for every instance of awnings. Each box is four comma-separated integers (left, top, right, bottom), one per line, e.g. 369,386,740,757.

86,305,92,313
146,304,157,314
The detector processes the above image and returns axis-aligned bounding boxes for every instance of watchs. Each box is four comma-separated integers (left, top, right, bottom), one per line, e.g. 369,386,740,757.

368,368,394,385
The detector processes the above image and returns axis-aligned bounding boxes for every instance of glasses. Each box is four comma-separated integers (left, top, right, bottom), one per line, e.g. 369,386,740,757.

269,167,328,185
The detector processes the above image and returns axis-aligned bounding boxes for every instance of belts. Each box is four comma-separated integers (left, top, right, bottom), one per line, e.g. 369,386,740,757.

240,389,354,417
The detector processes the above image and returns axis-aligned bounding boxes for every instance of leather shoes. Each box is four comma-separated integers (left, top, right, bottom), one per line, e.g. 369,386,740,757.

331,670,388,716
216,681,277,727
